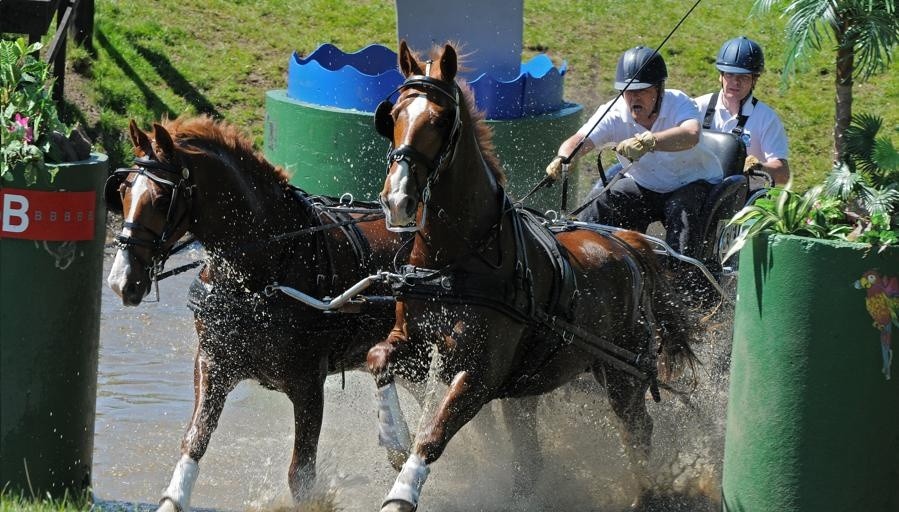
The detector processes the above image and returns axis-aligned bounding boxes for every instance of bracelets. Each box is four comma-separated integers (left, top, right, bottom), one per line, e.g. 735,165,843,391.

552,155,569,164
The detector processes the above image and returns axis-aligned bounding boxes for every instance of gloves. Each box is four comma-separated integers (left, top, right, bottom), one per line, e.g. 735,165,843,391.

615,130,658,160
546,156,569,180
743,155,763,174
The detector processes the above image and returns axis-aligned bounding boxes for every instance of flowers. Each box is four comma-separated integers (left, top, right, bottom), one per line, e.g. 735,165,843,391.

719,187,899,265
0,37,59,189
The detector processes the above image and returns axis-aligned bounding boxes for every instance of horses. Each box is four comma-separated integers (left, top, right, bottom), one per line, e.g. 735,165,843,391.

366,38,714,511
106,111,498,511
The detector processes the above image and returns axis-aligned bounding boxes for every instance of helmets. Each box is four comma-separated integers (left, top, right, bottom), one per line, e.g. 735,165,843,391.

716,36,764,74
614,46,668,89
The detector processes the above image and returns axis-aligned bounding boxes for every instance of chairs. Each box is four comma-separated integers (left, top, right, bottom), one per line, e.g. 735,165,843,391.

584,129,775,293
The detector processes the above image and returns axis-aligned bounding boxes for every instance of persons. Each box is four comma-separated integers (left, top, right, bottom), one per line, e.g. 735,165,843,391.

546,46,724,310
691,36,791,277
